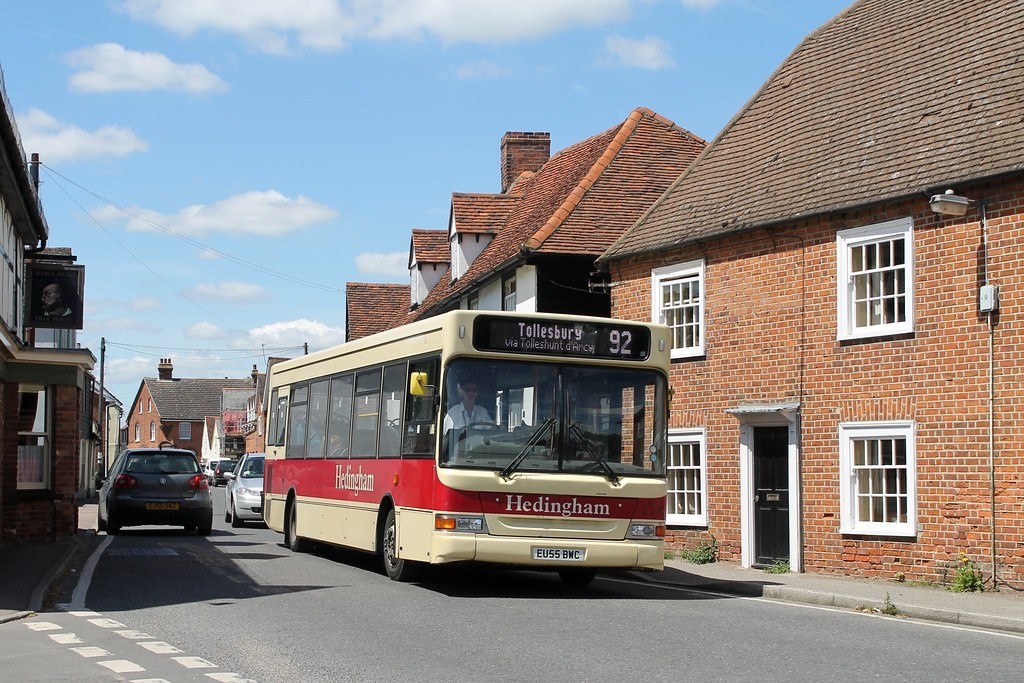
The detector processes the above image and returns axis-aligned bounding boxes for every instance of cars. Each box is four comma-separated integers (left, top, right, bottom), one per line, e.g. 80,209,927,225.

224,453,262,526
201,457,238,486
95,446,213,535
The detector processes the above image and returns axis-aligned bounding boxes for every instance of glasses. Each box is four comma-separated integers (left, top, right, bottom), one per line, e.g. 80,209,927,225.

460,386,479,393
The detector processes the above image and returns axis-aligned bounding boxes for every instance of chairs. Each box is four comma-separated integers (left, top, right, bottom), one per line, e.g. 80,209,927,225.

294,411,401,456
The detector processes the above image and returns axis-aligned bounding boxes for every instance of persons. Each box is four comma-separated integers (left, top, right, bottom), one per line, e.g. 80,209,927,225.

442,380,494,436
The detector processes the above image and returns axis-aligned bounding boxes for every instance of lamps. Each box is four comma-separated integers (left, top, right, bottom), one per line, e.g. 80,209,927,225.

928,194,980,216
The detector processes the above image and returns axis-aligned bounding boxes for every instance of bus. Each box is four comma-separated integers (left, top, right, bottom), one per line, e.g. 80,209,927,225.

263,309,673,583
